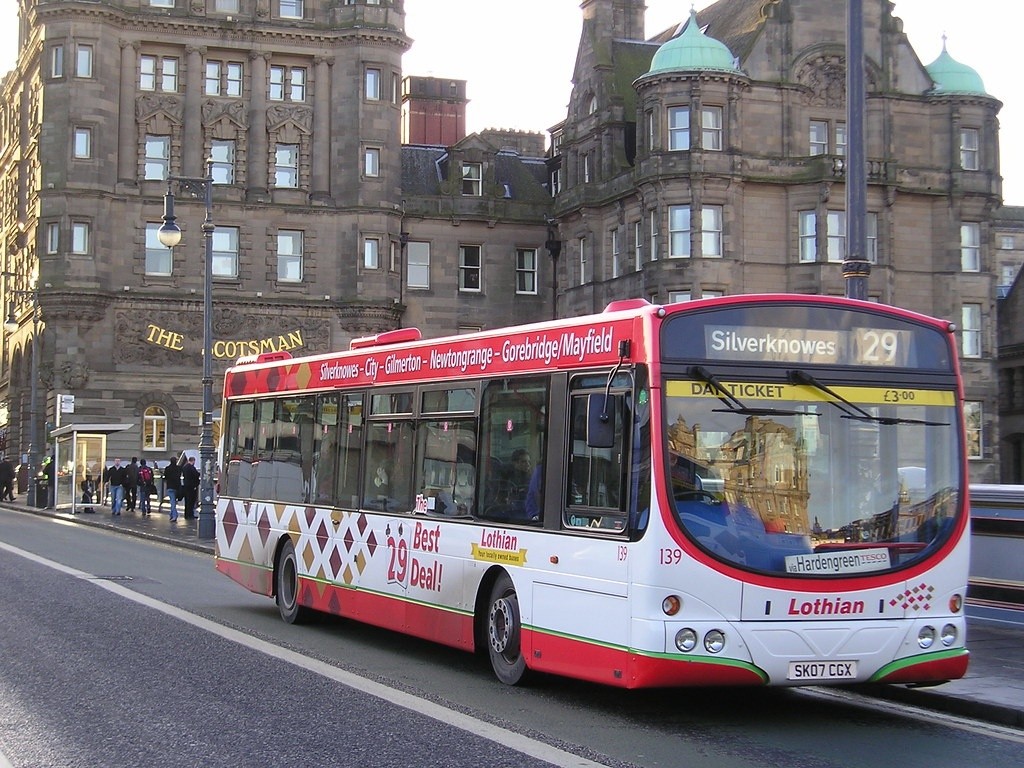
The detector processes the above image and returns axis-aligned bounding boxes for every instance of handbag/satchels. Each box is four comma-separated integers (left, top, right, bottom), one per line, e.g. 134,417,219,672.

150,484,157,495
177,485,185,501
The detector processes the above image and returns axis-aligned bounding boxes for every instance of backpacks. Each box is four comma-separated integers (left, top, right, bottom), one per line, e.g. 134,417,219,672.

140,466,152,482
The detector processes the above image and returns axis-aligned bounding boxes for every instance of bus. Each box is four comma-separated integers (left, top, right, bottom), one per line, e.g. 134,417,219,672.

211,292,972,688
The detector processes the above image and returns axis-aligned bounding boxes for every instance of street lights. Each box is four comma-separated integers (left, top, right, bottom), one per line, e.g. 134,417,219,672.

156,152,217,540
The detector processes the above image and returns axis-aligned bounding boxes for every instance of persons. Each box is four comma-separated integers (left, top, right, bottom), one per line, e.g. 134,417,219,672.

126,457,138,512
164,457,181,522
43,454,55,509
491,450,542,520
76,458,109,512
183,457,201,519
138,459,154,516
108,458,126,515
0,456,16,502
154,461,158,469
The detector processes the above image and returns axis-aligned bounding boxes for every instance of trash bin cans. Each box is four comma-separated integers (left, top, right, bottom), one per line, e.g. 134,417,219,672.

35,479,48,508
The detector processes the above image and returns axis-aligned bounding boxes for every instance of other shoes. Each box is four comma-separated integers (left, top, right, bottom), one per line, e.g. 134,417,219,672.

130,509,135,512
185,516,198,519
170,517,176,522
44,507,52,509
142,512,151,516
126,505,132,511
11,498,16,501
